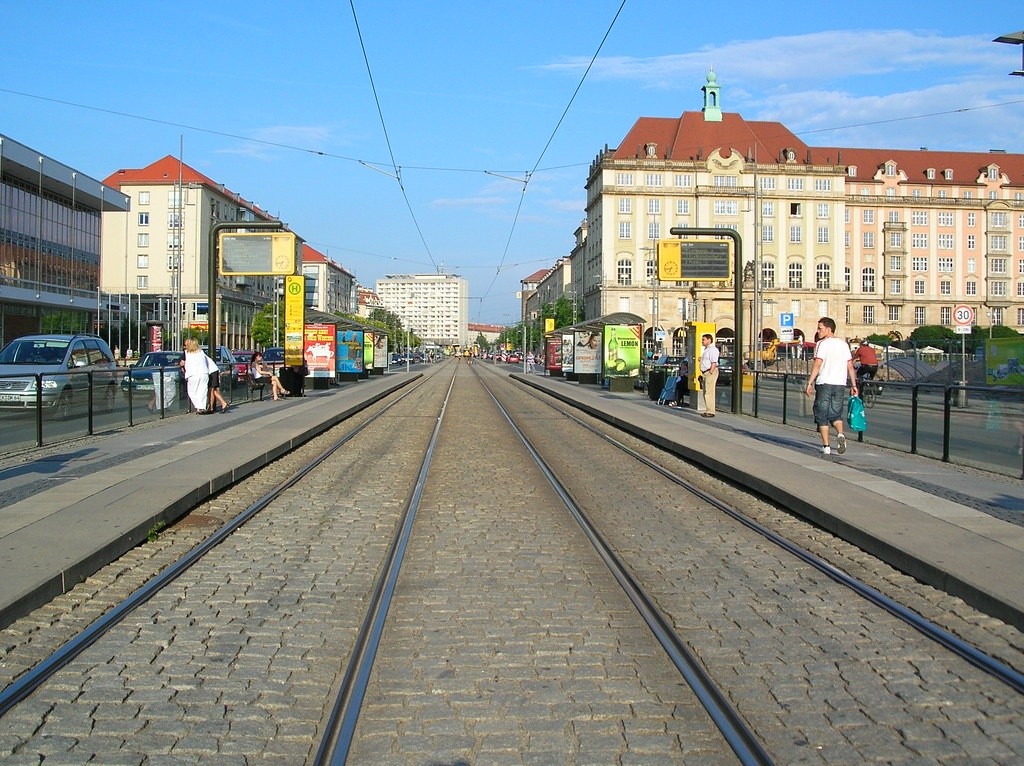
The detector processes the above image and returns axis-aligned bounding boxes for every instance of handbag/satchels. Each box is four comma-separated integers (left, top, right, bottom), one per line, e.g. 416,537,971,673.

847,395,867,431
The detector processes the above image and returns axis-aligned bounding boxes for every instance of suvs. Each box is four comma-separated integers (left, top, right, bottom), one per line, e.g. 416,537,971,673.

657,355,686,376
0,333,117,420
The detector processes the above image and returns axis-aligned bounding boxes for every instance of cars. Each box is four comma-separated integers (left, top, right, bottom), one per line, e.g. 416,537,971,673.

121,351,190,400
391,354,402,365
717,357,734,385
507,354,520,364
200,346,238,393
232,351,262,385
261,347,284,379
404,354,421,364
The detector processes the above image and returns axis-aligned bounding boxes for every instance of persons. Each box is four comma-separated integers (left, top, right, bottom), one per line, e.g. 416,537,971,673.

526,351,535,374
853,340,878,381
172,359,179,366
576,331,601,350
700,334,719,418
806,317,858,455
668,361,688,407
341,333,361,360
172,337,230,415
250,352,290,401
375,335,387,367
145,372,177,414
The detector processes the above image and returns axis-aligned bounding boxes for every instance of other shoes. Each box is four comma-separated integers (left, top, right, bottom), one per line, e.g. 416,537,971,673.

219,404,230,414
669,401,677,406
701,413,714,417
281,388,289,394
274,397,283,401
206,411,214,414
196,411,205,415
820,447,830,454
837,434,847,454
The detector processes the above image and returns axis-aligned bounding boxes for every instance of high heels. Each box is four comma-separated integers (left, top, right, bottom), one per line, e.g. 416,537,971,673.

145,404,153,415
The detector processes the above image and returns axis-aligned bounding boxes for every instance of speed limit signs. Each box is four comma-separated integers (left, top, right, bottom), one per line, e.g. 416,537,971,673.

953,305,974,326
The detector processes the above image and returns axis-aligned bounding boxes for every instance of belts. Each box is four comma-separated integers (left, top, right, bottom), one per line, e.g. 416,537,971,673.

702,369,710,373
861,364,878,367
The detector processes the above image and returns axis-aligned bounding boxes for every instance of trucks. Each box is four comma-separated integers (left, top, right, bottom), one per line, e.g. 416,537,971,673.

747,341,815,367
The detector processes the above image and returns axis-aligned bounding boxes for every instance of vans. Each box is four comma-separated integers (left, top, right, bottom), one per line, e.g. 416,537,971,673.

514,352,524,361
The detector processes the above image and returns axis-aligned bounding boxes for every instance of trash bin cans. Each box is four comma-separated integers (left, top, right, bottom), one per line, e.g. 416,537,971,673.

279,366,303,397
649,364,665,400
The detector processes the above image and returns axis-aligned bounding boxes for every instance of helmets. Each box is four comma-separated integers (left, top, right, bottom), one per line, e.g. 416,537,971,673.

860,340,869,346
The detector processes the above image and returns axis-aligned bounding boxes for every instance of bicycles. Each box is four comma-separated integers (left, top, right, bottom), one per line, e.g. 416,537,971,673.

845,365,876,408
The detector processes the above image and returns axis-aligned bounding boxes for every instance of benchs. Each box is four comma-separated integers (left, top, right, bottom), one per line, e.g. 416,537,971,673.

246,374,266,402
